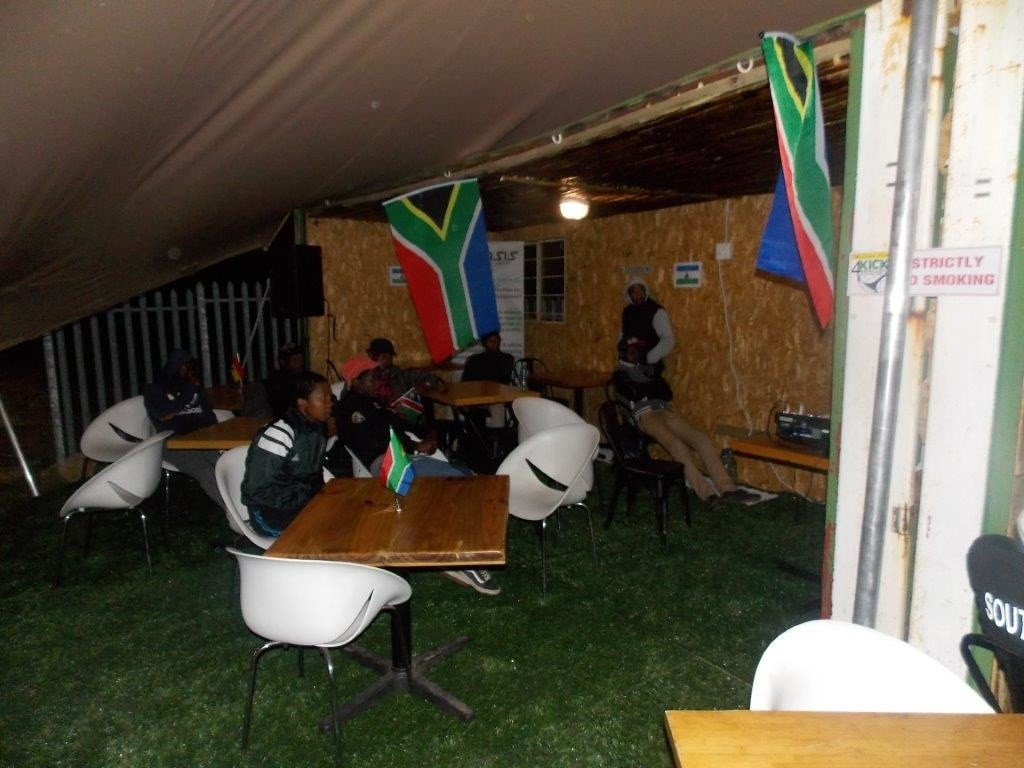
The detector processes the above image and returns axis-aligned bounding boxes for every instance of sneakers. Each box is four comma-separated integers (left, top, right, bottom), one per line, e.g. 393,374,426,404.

445,570,502,595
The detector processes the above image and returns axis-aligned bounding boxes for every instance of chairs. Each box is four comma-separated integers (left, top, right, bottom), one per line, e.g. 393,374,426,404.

50,357,691,768
750,619,996,715
960,533,1024,714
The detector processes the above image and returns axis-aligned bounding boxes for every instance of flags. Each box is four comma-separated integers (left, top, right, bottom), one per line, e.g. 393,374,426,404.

376,431,415,497
382,179,501,364
393,388,423,418
229,350,246,388
755,31,836,331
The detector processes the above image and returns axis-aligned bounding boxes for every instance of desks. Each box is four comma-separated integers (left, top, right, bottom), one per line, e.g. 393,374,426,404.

402,362,465,382
731,434,828,471
664,708,1024,768
168,418,335,549
413,379,539,468
527,368,614,418
264,473,511,732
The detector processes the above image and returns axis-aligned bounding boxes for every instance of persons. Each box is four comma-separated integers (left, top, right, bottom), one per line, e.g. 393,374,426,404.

610,336,762,512
142,348,248,535
241,371,333,536
268,341,320,398
617,277,674,376
328,382,503,595
460,330,515,463
351,337,432,440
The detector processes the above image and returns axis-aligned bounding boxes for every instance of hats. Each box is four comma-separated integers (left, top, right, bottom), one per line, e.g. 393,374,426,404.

617,336,646,349
369,337,397,357
342,356,381,388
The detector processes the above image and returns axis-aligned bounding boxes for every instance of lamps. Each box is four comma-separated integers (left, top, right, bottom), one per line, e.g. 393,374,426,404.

559,196,591,221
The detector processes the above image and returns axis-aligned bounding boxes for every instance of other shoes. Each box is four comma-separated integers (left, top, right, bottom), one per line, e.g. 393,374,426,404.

724,492,762,502
706,495,722,513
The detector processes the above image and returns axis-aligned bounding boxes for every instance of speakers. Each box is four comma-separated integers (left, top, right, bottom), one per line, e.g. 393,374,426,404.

270,244,325,319
